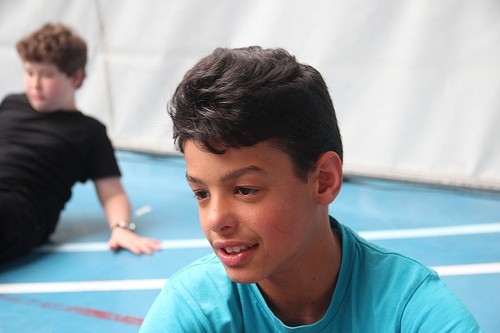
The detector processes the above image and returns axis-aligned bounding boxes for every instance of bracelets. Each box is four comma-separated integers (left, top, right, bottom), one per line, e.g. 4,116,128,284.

109,222,136,231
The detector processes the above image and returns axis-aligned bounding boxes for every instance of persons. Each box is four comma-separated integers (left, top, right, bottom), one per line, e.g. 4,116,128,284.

136,46,482,333
0,21,162,273
